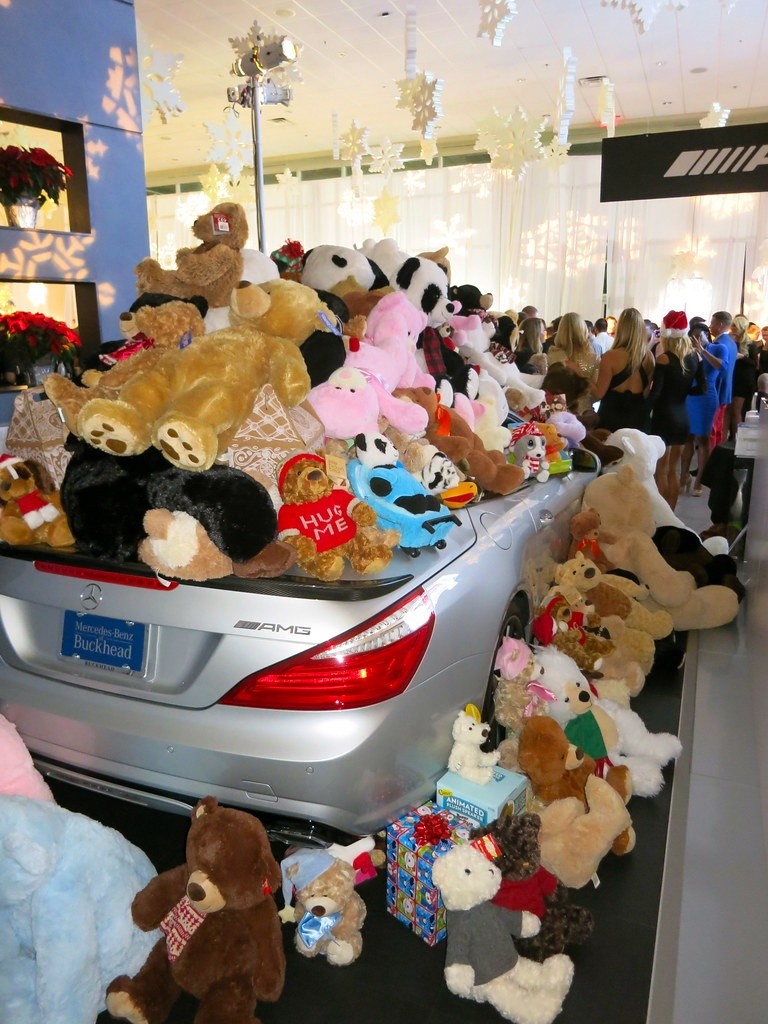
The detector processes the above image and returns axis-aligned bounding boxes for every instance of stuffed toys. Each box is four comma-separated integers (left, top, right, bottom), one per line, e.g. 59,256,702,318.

105,795,286,1024
467,813,593,962
0,198,626,587
277,850,368,966
1,794,170,1024
444,427,749,903
429,846,575,1024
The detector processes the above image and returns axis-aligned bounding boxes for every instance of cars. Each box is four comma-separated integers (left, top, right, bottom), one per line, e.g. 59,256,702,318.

0,385,609,854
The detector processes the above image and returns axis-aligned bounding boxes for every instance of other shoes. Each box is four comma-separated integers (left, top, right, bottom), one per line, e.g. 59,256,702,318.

689,468,698,476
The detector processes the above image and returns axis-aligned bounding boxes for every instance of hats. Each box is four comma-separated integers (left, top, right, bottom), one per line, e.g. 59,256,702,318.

661,309,690,337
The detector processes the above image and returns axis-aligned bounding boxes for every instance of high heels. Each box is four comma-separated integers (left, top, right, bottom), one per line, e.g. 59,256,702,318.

677,475,692,493
691,486,703,496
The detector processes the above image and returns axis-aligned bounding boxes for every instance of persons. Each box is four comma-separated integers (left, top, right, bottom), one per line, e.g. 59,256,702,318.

482,301,767,512
